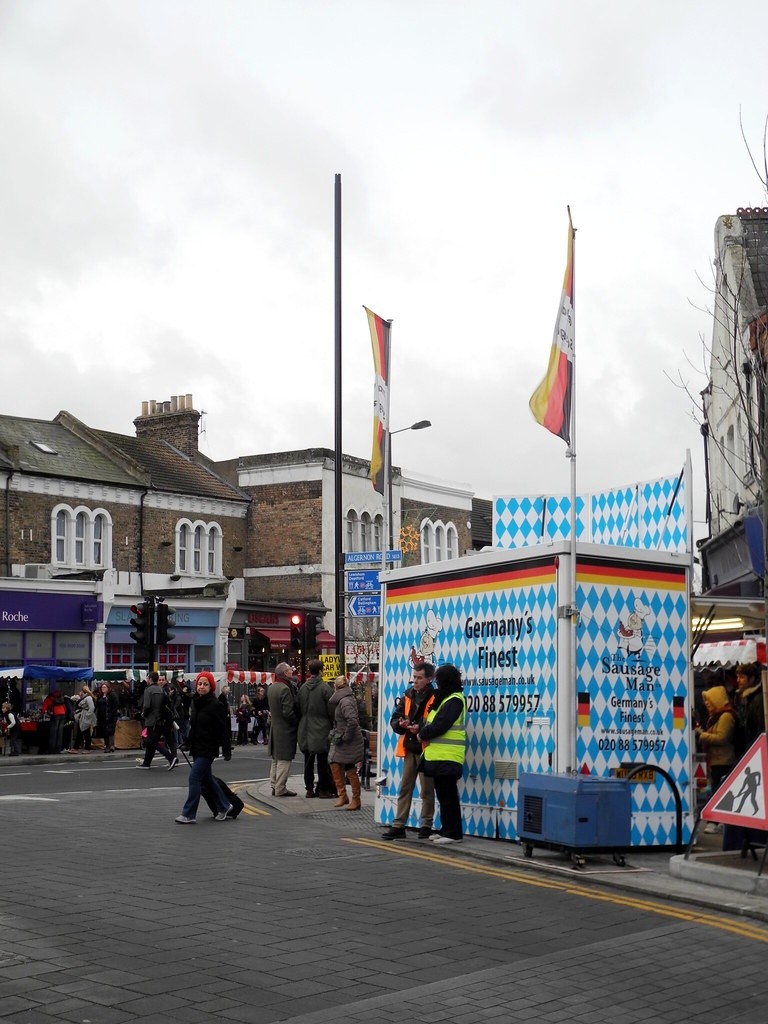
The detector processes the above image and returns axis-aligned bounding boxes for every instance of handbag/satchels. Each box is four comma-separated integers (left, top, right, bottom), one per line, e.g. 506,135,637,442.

160,689,171,720
328,729,345,745
91,726,103,739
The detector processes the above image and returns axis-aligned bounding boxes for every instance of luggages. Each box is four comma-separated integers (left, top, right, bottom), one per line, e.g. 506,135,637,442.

179,744,244,820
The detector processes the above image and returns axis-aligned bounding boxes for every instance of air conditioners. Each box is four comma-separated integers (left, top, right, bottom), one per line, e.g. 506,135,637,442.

25,564,53,579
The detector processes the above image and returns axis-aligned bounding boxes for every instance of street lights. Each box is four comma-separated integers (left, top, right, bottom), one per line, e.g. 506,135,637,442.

386,420,436,571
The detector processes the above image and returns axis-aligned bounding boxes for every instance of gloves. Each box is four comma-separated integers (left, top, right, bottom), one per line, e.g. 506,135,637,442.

224,756,231,761
181,744,189,752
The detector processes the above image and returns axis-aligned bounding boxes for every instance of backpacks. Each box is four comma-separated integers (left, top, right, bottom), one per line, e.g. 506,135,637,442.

52,697,65,705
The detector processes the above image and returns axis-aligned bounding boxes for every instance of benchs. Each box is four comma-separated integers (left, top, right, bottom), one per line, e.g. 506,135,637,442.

361,729,377,790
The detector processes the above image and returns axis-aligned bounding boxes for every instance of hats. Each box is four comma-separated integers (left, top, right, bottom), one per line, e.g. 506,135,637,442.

194,671,216,692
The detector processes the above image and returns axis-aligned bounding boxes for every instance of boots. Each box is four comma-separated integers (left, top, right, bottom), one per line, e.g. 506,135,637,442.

253,739,257,745
329,762,349,807
263,738,268,745
345,767,361,812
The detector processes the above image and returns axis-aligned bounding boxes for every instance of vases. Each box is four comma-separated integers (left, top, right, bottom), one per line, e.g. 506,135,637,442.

171,576,181,581
233,547,242,551
226,576,235,580
162,542,172,546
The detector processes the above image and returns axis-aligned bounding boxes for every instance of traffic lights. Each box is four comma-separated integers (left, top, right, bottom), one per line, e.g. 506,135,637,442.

290,614,303,650
129,603,149,646
156,603,176,646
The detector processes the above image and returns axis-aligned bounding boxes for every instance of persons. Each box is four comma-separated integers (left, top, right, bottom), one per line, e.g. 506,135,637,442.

383,663,435,842
690,661,764,835
417,665,466,844
0,659,377,811
174,671,233,824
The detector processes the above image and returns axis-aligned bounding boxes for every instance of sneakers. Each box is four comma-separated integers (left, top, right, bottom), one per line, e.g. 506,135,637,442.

703,823,720,834
175,815,196,823
215,804,233,820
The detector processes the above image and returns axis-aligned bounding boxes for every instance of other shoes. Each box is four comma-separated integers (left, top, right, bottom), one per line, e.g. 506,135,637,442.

279,791,297,796
230,741,247,750
418,827,433,839
271,789,275,795
168,757,177,771
104,746,115,753
319,792,337,798
433,837,463,844
8,751,19,756
429,834,442,841
172,750,179,763
136,764,149,770
381,826,406,838
60,748,91,754
306,790,314,798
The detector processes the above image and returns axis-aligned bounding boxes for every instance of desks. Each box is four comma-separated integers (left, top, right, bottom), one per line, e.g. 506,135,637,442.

231,717,256,741
